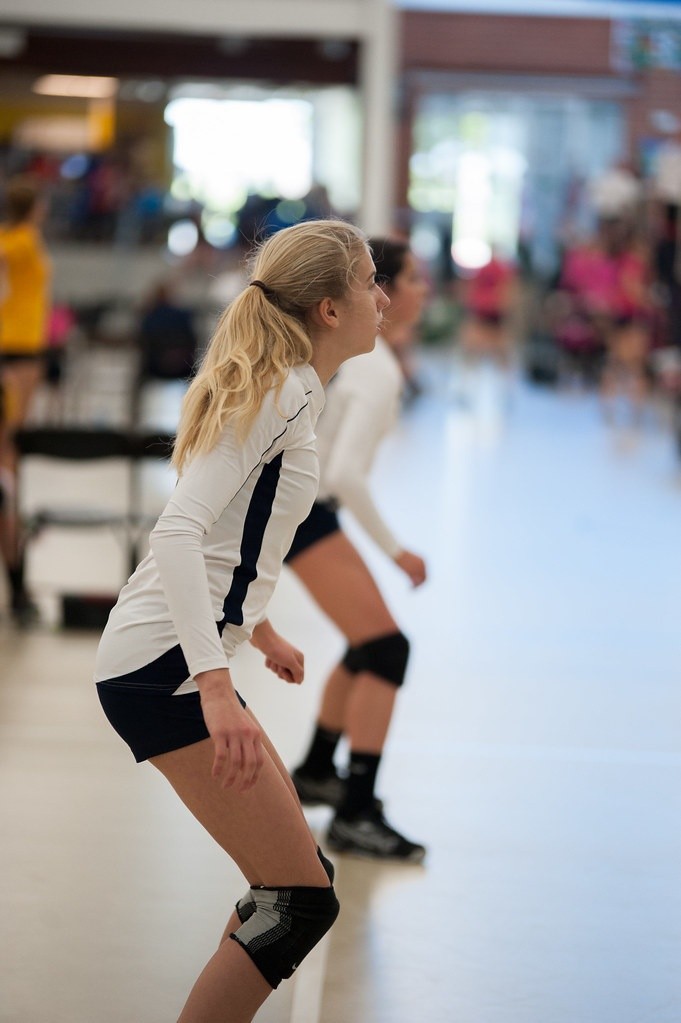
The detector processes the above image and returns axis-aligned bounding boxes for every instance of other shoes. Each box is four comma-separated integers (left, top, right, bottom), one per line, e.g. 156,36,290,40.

326,818,426,867
292,770,348,805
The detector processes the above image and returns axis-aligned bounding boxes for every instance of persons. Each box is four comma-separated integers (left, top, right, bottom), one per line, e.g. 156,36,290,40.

0,170,52,626
282,238,427,863
93,221,391,1023
458,226,658,407
